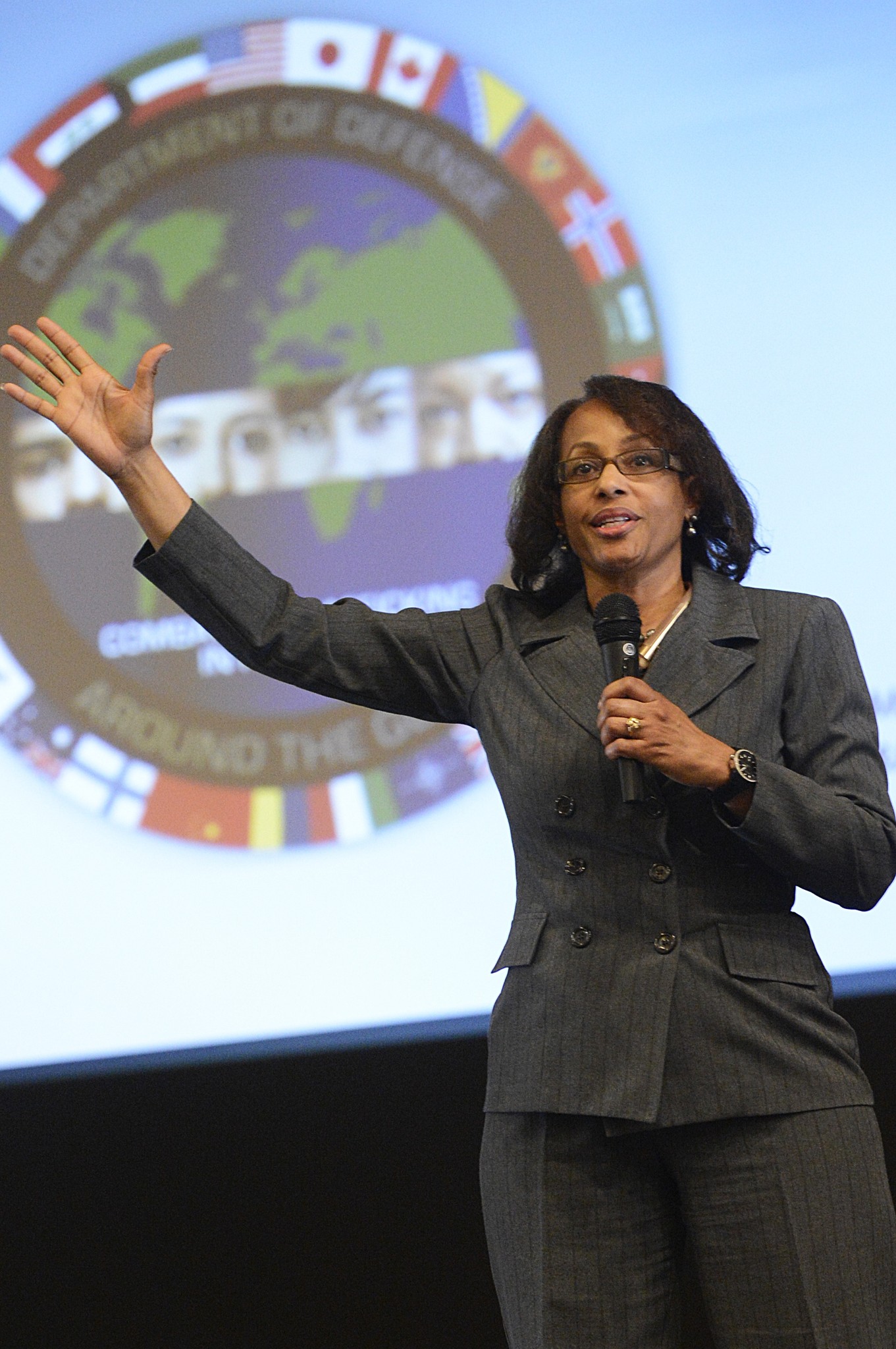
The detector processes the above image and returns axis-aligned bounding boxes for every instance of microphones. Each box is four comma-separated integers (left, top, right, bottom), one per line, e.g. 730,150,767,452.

594,593,647,805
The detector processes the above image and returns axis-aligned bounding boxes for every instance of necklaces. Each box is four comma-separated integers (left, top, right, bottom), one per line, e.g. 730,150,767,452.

636,628,654,645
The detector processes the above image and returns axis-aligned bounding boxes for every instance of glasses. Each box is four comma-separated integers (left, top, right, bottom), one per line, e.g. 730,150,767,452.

553,446,689,483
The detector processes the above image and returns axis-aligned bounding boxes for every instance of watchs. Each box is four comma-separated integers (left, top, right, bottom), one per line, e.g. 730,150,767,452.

708,743,757,808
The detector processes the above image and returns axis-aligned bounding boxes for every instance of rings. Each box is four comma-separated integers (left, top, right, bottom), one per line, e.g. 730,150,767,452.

627,715,637,733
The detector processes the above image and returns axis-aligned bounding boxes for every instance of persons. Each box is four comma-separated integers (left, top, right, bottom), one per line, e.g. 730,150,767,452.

0,313,896,1349
